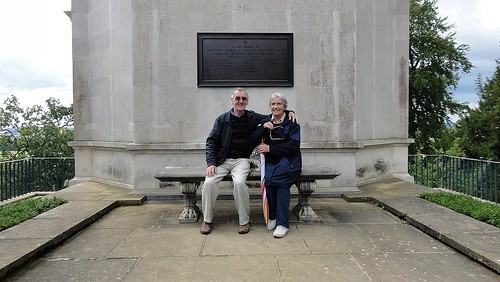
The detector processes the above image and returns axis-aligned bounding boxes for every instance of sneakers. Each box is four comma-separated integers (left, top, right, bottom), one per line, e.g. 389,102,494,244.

268,219,276,230
239,222,249,233
200,222,212,234
273,225,289,237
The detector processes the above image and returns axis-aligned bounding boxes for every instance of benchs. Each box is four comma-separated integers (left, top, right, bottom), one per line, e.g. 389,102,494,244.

153,166,342,223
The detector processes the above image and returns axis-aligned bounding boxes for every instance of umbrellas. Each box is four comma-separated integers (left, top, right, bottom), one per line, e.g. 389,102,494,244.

256,124,269,233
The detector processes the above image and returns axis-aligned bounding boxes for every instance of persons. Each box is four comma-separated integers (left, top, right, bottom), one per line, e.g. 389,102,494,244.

200,88,298,234
253,93,301,238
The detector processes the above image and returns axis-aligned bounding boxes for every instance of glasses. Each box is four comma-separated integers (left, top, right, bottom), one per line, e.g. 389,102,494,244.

235,96,247,101
270,126,284,140
